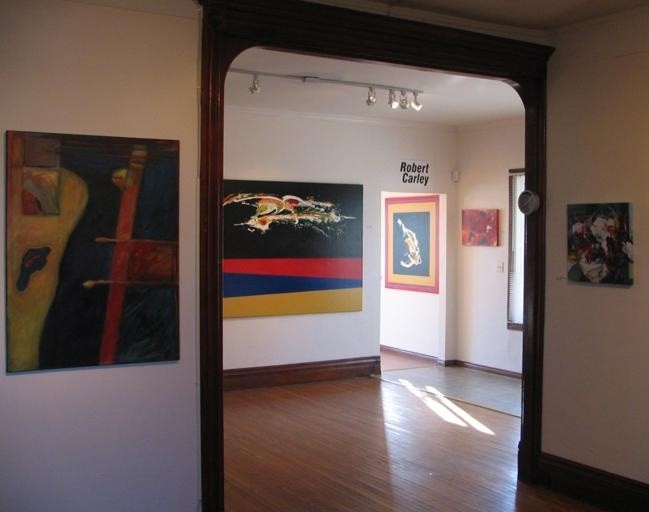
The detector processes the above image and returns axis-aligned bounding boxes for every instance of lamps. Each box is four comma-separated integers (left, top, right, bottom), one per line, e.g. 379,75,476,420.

249,72,422,111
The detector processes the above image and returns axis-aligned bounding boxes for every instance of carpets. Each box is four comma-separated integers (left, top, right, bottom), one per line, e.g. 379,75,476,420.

371,365,522,418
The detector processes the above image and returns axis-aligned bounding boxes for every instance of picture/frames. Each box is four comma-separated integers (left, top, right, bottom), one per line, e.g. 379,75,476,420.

385,195,439,294
